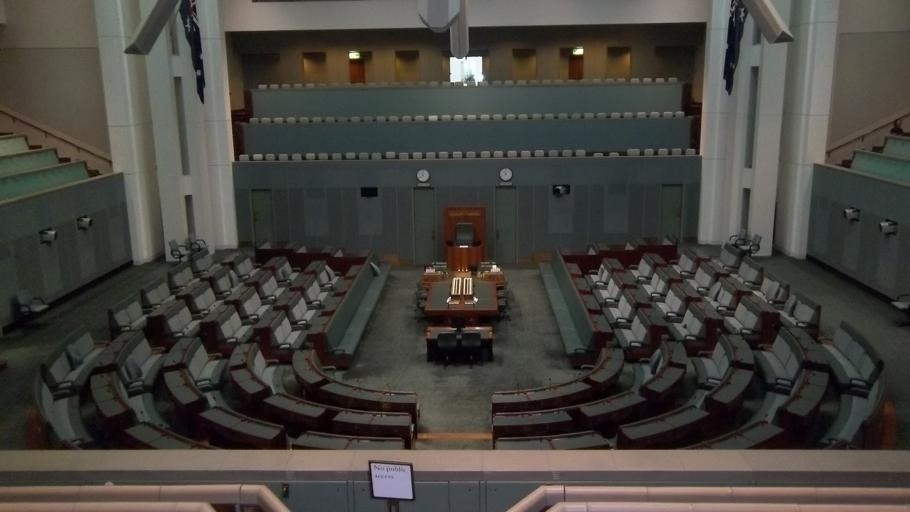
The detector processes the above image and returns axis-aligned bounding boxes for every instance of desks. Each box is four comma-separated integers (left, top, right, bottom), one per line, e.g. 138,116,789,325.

424,279,498,324
427,327,494,362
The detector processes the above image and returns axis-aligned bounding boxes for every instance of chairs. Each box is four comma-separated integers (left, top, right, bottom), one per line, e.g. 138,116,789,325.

15,288,48,333
185,238,207,255
729,229,747,246
890,293,910,327
461,332,483,366
434,333,457,368
740,233,762,257
168,240,188,266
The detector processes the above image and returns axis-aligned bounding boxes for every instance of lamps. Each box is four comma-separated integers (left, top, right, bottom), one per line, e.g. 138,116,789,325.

39,228,57,246
878,219,897,236
843,207,860,222
552,184,570,196
77,214,93,230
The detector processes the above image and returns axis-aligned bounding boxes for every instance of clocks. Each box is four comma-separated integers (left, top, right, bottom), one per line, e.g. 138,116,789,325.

416,169,430,183
499,167,513,182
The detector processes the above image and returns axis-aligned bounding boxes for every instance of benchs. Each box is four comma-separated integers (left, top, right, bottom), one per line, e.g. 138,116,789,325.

847,122,909,187
0,128,91,201
